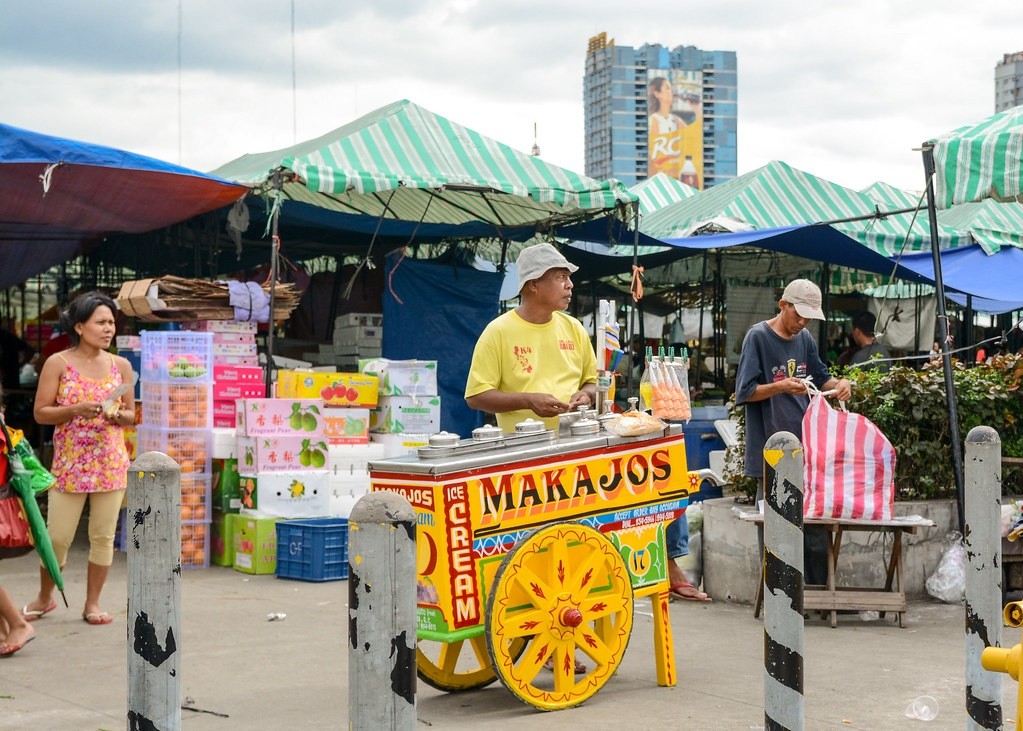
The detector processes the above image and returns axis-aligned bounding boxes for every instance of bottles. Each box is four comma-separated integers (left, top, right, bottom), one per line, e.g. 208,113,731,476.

679,156,698,189
673,93,700,103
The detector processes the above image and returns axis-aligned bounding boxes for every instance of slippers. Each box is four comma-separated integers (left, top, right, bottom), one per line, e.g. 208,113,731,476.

0,634,36,657
83,612,112,624
670,584,712,602
19,600,55,622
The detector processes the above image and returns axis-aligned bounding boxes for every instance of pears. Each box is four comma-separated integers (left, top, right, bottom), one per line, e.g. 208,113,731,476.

168,357,205,377
300,445,324,468
289,410,317,431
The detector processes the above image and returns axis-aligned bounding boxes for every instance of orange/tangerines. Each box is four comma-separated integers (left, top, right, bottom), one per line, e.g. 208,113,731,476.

146,385,208,567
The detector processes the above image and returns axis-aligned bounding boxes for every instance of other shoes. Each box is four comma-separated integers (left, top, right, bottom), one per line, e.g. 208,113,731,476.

542,654,586,673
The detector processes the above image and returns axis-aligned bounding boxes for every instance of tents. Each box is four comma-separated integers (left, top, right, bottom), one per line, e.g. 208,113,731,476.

0,98,1022,412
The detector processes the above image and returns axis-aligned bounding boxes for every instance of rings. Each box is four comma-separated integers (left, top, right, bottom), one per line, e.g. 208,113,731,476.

96,408,99,413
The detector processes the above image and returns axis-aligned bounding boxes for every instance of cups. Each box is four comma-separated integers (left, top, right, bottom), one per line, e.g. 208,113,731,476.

102,401,119,417
640,381,655,409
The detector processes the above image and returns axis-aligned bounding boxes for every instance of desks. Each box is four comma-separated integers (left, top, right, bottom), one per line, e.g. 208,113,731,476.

731,505,939,628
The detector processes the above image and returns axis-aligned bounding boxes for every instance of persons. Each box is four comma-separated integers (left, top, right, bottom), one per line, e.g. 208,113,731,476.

665,511,712,603
18,295,135,624
0,583,34,656
464,243,600,432
735,278,853,564
848,311,891,373
930,342,943,370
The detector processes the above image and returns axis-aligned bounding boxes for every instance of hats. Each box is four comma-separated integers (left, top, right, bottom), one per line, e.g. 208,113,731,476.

517,243,579,294
782,278,827,321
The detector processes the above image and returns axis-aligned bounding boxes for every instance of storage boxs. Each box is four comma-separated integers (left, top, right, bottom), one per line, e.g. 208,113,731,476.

112,312,445,586
691,405,728,420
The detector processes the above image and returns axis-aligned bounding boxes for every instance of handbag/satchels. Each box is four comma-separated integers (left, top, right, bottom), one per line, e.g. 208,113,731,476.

0,425,63,560
802,391,896,521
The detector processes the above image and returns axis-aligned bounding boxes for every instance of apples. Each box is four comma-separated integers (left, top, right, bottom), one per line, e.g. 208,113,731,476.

321,384,358,401
